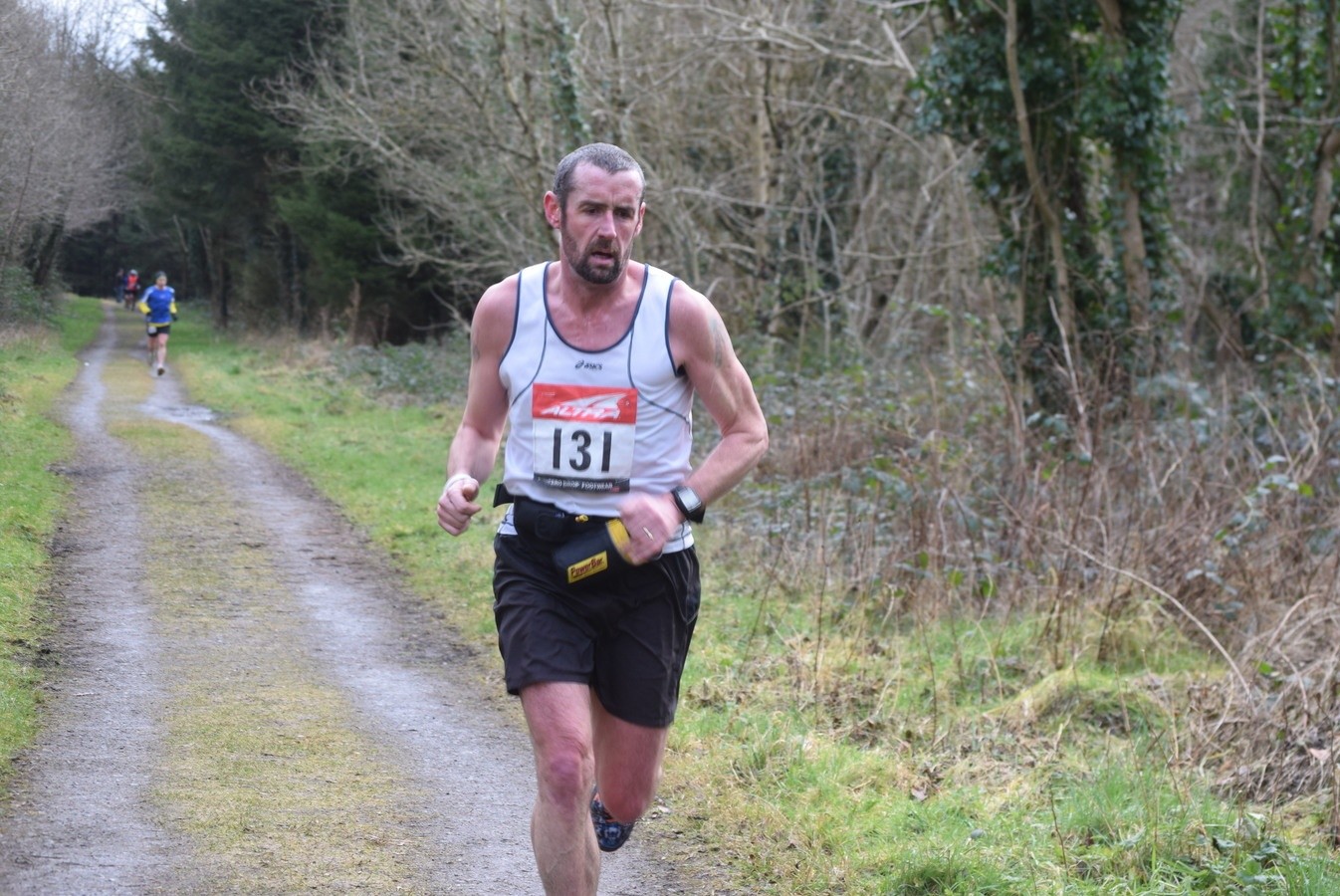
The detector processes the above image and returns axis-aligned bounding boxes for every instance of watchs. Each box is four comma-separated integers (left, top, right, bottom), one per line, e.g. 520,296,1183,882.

670,484,706,522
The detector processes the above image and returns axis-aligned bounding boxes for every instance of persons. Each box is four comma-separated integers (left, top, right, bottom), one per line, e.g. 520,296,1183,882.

123,270,140,311
139,271,178,375
115,268,126,303
434,138,770,895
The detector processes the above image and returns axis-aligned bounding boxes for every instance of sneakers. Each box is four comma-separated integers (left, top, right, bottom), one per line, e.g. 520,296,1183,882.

586,787,643,855
